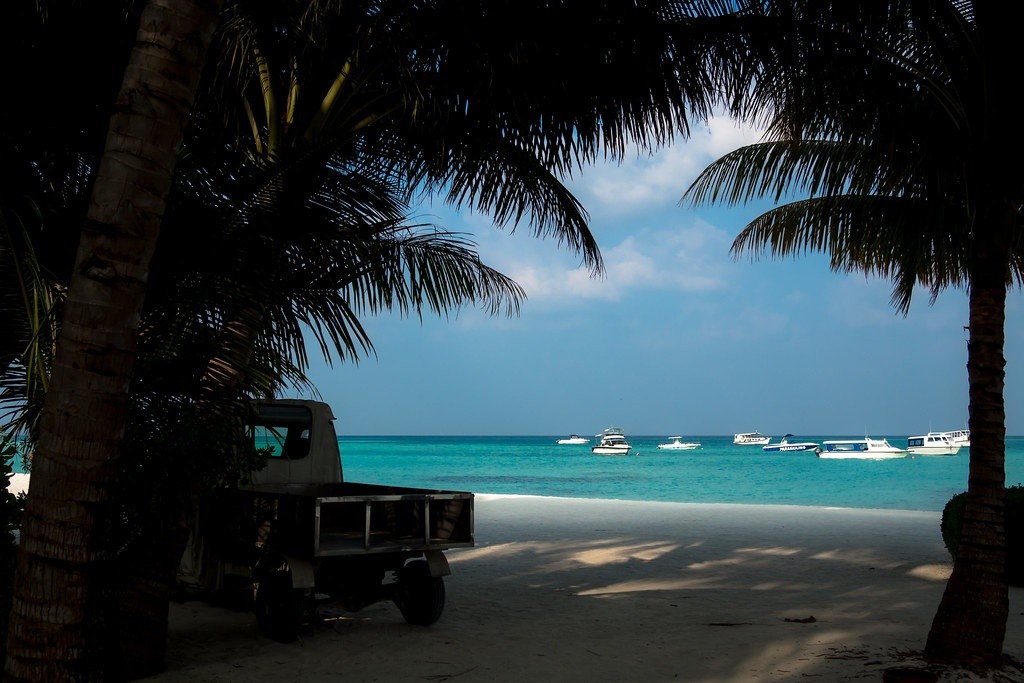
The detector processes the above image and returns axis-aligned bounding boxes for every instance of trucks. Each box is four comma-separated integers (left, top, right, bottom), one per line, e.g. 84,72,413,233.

137,398,477,645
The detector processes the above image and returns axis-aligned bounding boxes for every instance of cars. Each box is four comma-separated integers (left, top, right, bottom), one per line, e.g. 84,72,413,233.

656,436,701,450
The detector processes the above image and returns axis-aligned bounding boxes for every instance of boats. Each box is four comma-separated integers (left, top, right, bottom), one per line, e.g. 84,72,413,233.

591,427,632,456
762,436,820,451
556,435,591,445
907,421,970,456
732,432,772,445
818,438,908,461
